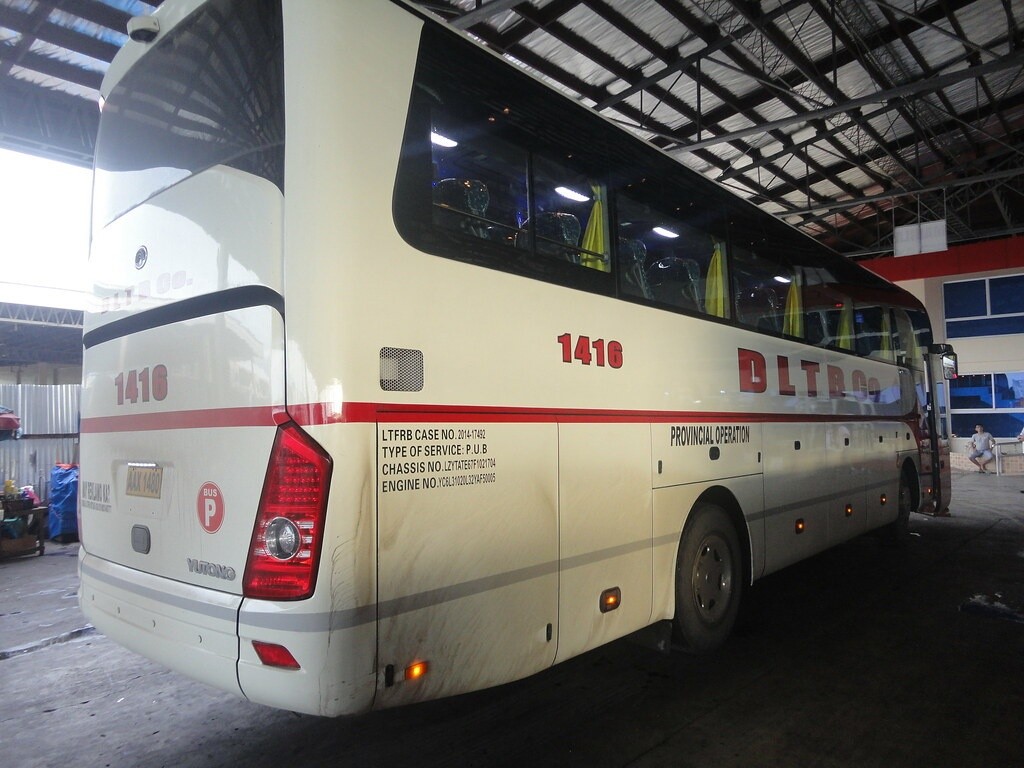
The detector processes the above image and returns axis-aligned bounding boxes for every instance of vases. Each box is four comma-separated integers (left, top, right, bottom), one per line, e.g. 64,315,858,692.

2,498,22,512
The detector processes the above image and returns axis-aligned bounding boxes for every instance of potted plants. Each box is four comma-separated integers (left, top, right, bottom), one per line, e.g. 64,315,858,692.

19,491,34,509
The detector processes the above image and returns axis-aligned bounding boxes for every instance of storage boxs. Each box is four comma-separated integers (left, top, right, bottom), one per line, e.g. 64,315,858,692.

0,536,36,552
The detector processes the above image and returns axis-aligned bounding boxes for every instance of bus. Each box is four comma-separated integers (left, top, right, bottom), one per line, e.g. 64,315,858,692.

77,1,961,719
948,374,1015,411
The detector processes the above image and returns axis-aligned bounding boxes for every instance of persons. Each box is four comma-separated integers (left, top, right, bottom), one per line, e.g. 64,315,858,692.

1017,427,1024,493
968,424,996,473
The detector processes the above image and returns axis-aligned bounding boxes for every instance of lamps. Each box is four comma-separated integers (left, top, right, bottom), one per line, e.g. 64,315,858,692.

774,269,792,283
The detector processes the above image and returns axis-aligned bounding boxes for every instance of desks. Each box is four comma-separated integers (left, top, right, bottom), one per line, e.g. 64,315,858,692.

994,442,1024,477
0,506,48,556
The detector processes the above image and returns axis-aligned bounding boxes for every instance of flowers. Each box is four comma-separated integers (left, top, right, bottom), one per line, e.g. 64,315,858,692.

3,480,18,499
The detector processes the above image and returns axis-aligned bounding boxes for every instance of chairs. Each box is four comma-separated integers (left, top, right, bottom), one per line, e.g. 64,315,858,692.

516,211,581,263
735,287,778,332
432,178,490,240
805,303,837,346
619,234,647,299
853,323,880,356
646,256,701,311
